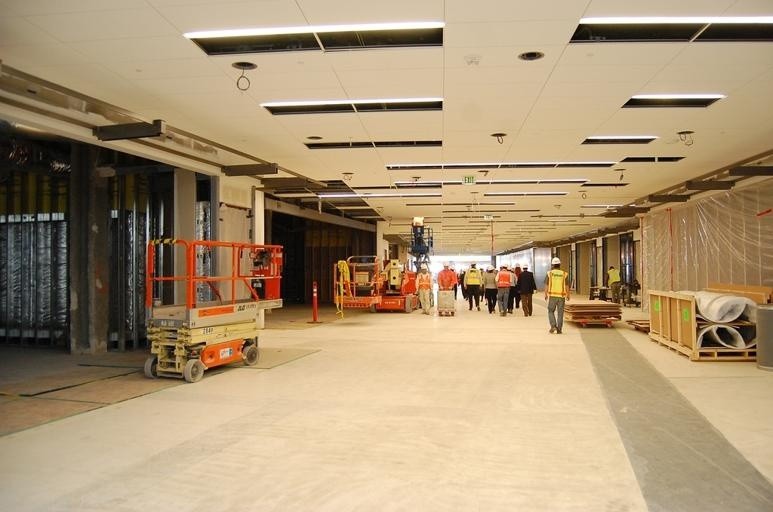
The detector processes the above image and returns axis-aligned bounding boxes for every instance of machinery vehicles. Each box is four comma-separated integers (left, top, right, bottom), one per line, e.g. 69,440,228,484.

332,255,420,312
144,237,284,385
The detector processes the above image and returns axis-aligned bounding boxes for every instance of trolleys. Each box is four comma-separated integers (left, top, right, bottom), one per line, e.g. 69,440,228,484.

589,285,611,301
437,287,455,316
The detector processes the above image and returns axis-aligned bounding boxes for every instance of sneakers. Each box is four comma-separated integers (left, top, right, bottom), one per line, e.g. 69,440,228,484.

550,327,562,334
477,303,532,317
421,310,430,315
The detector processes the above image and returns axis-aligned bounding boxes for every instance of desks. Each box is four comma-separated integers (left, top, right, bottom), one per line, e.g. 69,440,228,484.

589,287,637,303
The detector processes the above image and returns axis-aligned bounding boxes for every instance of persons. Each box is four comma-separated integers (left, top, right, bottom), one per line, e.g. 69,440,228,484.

437,262,538,317
544,257,570,334
605,266,621,304
415,264,432,315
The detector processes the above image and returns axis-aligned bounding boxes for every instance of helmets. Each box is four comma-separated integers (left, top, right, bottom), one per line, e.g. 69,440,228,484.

442,260,448,266
552,257,560,264
463,261,528,272
421,264,427,270
608,265,615,270
450,265,454,270
